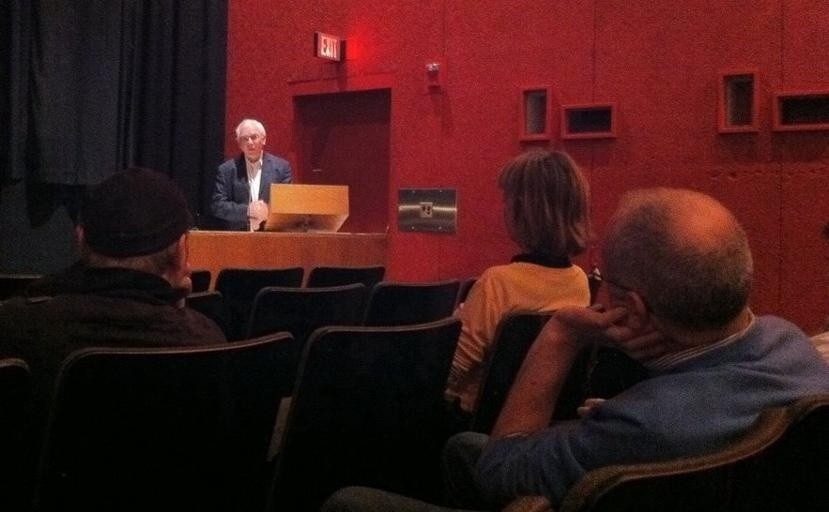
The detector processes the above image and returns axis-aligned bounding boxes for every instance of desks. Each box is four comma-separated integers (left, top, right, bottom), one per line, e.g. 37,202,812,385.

187,230,388,291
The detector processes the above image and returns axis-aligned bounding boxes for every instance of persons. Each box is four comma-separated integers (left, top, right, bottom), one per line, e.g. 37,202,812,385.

445,146,593,425
213,118,290,234
0,165,230,372
454,179,829,511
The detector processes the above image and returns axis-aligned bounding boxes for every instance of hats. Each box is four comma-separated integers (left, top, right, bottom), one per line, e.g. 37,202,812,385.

83,167,197,257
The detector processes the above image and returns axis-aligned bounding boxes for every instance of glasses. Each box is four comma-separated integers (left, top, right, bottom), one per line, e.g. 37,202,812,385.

588,266,656,316
